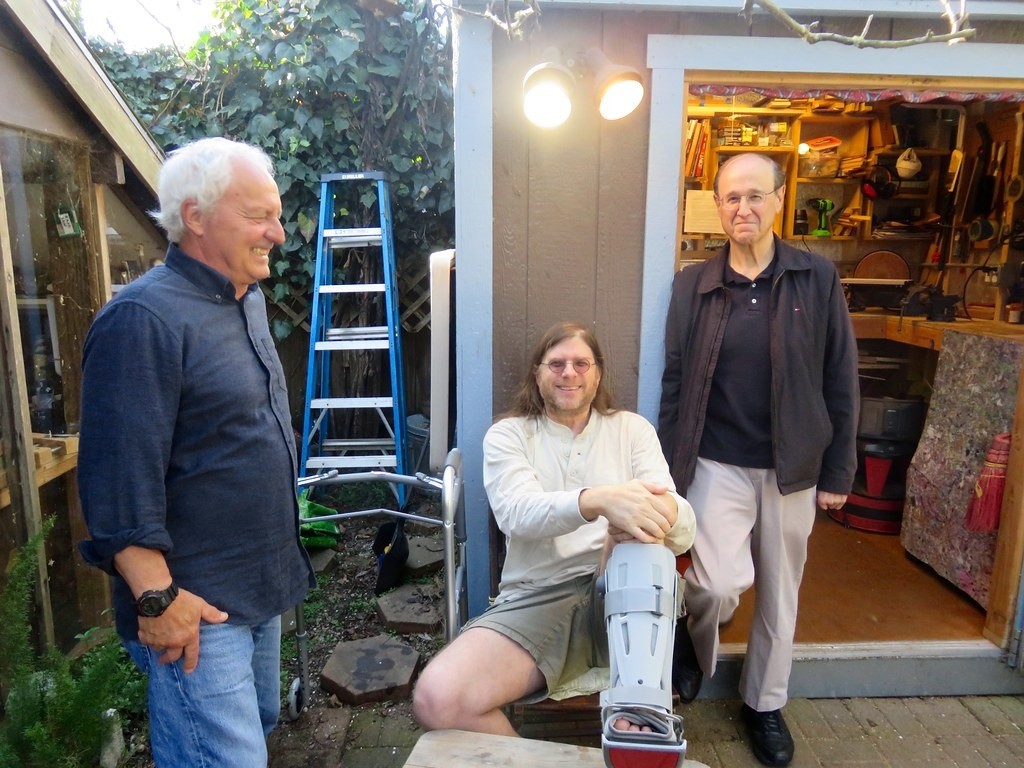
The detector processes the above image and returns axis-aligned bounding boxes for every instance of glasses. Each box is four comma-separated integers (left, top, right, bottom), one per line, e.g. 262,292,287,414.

540,358,596,374
717,184,783,205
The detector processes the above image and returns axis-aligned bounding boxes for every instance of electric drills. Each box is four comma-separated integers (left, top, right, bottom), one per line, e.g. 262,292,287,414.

809,198,835,237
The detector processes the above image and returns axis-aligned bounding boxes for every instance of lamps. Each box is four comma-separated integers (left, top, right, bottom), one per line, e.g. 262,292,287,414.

587,47,643,120
522,48,575,128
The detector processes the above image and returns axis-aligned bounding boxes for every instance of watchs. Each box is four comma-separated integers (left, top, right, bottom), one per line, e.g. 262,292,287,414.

137,578,180,617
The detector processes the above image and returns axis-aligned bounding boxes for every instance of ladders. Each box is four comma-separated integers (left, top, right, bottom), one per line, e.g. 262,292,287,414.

293,171,413,513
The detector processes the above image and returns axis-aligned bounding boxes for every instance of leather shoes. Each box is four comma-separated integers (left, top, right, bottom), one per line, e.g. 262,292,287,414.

742,702,795,768
674,618,703,704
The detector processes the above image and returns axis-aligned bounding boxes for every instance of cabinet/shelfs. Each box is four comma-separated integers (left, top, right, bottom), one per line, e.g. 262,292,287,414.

675,73,1024,356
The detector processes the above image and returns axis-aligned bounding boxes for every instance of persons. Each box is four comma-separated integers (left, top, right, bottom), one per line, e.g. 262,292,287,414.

78,138,318,767
412,322,695,768
657,152,859,768
